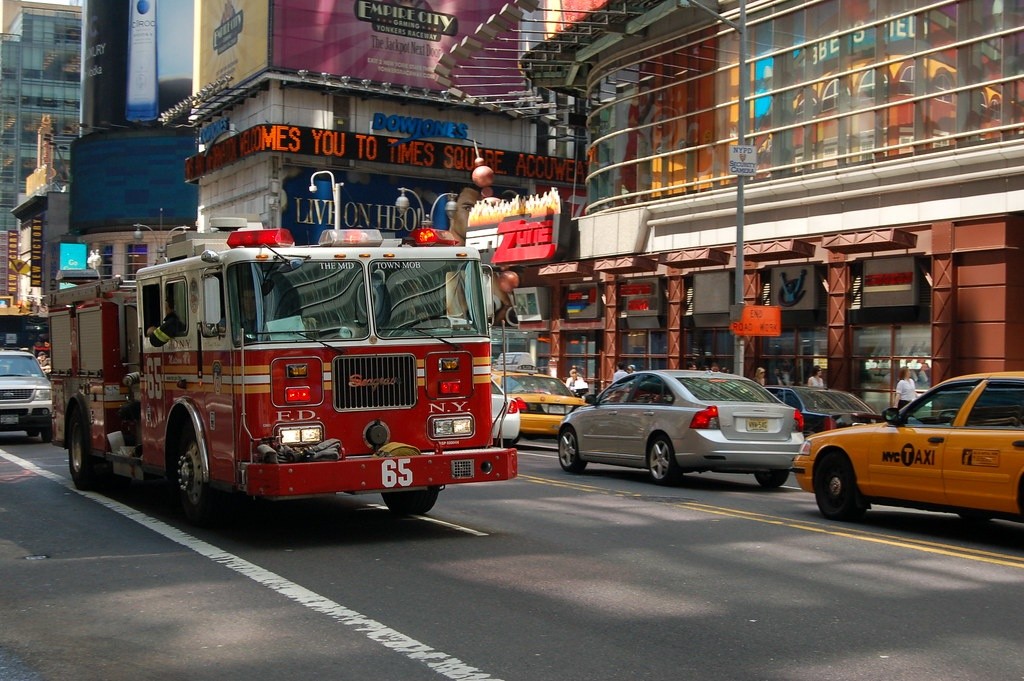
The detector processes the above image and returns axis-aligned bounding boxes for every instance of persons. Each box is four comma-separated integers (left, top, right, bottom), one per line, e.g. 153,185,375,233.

893,366,918,410
751,367,765,387
808,365,823,386
391,275,431,326
36,352,50,369
447,183,484,246
773,366,795,387
565,365,588,390
147,295,182,347
685,361,730,374
202,278,258,338
613,362,638,383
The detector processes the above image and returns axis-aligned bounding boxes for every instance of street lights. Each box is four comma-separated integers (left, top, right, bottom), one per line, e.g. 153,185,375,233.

133,207,190,257
395,187,459,228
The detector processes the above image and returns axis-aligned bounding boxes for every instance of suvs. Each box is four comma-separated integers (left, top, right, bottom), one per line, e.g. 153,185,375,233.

0,350,52,442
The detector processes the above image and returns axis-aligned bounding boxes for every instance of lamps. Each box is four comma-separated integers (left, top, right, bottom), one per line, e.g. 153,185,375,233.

362,79,372,87
319,71,331,83
556,138,588,142
341,76,350,86
43,121,129,150
422,0,670,128
537,135,585,141
559,133,588,137
298,69,309,81
382,81,391,92
403,85,411,95
157,74,249,130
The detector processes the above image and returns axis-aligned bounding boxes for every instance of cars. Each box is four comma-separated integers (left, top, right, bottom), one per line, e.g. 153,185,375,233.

556,368,804,489
760,385,884,440
489,379,520,447
490,372,586,437
789,370,1024,521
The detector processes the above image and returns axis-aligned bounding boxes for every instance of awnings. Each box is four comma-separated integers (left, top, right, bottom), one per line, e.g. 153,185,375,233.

10,195,47,223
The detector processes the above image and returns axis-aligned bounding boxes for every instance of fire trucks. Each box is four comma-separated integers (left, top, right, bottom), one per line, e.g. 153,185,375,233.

44,217,519,523
